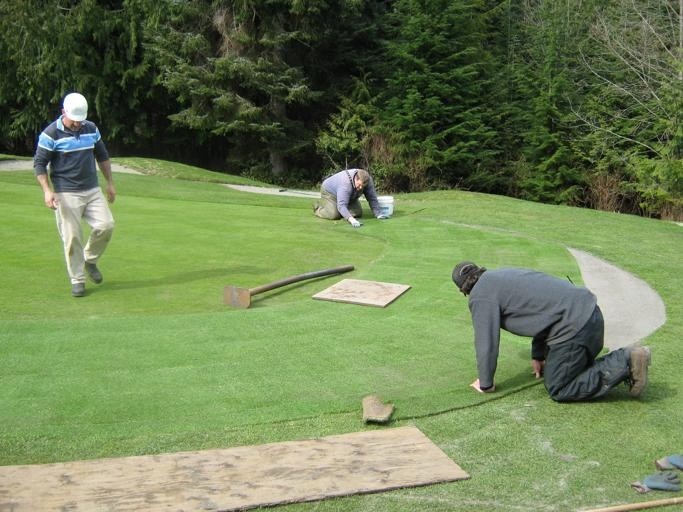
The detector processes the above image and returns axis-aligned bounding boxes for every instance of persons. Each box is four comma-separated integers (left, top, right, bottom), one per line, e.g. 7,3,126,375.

313,168,388,229
452,260,653,402
31,91,115,299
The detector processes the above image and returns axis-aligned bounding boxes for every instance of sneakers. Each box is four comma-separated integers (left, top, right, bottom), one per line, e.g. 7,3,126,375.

629,346,652,399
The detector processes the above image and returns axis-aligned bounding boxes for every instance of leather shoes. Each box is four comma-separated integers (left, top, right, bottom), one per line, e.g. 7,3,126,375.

71,283,84,297
84,261,102,283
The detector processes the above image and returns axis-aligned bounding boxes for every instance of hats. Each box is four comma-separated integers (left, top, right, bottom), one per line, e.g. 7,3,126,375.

452,261,479,287
62,93,88,122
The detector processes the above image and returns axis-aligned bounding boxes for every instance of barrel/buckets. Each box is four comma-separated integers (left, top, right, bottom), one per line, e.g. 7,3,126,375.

378,194,395,219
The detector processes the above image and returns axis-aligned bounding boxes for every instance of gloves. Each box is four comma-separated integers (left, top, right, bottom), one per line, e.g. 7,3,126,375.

347,216,363,228
655,453,683,470
630,470,681,493
376,214,388,219
